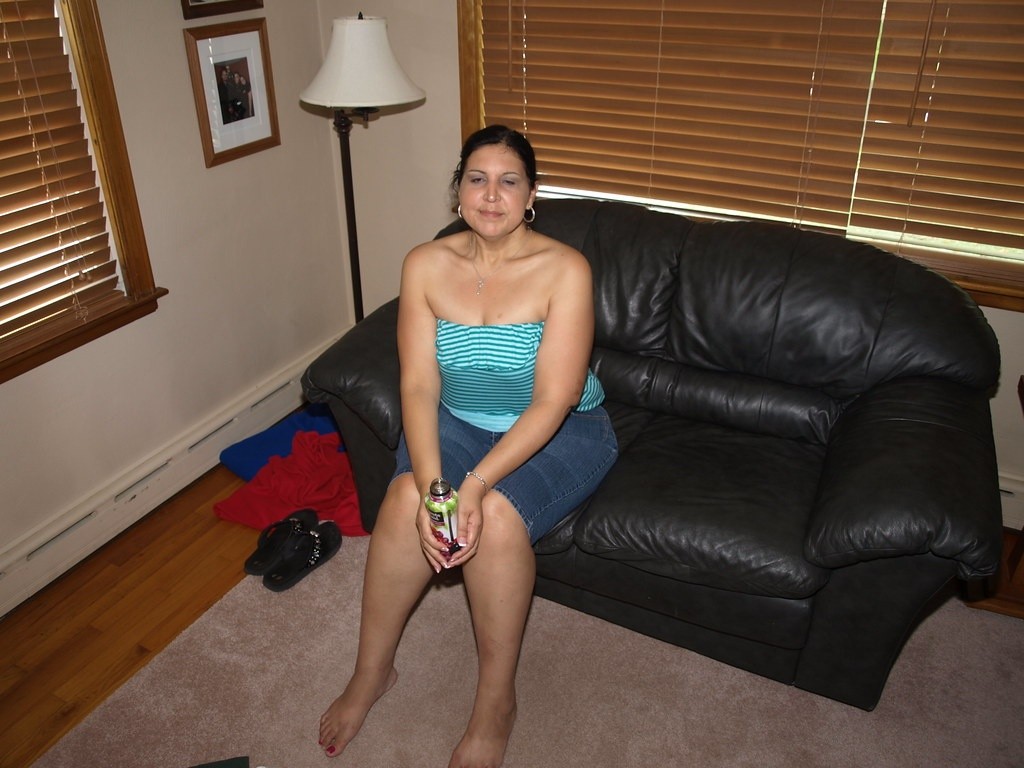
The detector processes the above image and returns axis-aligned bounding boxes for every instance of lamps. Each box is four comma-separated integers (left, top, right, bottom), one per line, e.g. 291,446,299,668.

298,12,426,333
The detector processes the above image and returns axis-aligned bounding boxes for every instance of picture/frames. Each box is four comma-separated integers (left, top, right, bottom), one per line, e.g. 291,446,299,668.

177,17,282,170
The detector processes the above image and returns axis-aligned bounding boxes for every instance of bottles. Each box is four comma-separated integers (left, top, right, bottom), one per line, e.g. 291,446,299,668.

424,477,463,563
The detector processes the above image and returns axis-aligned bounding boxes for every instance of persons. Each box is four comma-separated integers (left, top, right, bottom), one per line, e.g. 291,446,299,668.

216,62,252,124
317,123,627,768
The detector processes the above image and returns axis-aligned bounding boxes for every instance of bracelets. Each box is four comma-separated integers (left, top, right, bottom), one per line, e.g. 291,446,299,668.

465,471,489,494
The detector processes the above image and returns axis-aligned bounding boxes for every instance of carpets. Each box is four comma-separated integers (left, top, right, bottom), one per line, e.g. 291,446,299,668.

20,519,1022,768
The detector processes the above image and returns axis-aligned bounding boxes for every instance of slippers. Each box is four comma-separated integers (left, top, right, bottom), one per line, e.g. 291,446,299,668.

244,508,342,592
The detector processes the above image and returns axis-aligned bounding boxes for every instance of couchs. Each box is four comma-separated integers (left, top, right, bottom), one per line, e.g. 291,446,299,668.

296,183,1013,719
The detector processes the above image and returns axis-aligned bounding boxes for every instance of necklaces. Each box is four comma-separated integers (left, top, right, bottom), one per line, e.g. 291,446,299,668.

466,224,533,294
